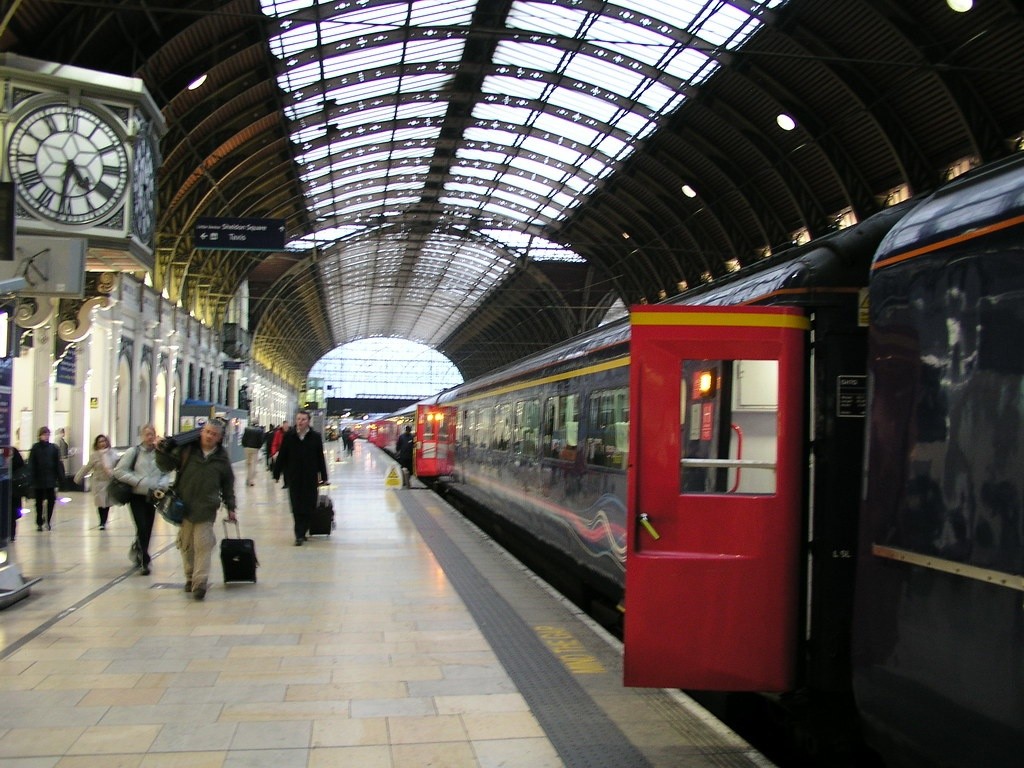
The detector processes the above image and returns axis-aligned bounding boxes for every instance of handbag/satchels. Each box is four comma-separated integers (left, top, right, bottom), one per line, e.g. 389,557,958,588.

111,477,132,505
158,489,185,523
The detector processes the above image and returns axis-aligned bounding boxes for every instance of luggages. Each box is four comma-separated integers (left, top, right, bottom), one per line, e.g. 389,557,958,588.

309,483,334,535
221,519,257,584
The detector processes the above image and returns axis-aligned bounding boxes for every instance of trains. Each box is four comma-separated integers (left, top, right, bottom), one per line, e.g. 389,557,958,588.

354,146,1024,768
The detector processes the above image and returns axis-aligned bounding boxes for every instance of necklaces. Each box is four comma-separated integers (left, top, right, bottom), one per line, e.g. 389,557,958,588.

114,424,177,575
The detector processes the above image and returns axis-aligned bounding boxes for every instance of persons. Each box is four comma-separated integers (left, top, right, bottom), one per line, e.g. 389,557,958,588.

154,418,237,599
326,424,354,456
241,418,265,486
8,428,67,541
264,421,294,483
75,435,119,530
396,425,416,488
239,384,252,416
272,411,328,546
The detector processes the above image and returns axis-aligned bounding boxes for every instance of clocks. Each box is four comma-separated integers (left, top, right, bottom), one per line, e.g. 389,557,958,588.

134,128,157,240
0,78,135,230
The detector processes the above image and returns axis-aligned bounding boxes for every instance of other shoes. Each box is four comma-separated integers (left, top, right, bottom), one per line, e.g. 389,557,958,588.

194,588,205,599
140,561,149,574
185,581,191,591
38,527,42,531
296,536,307,546
100,524,104,529
132,543,142,563
48,522,51,530
